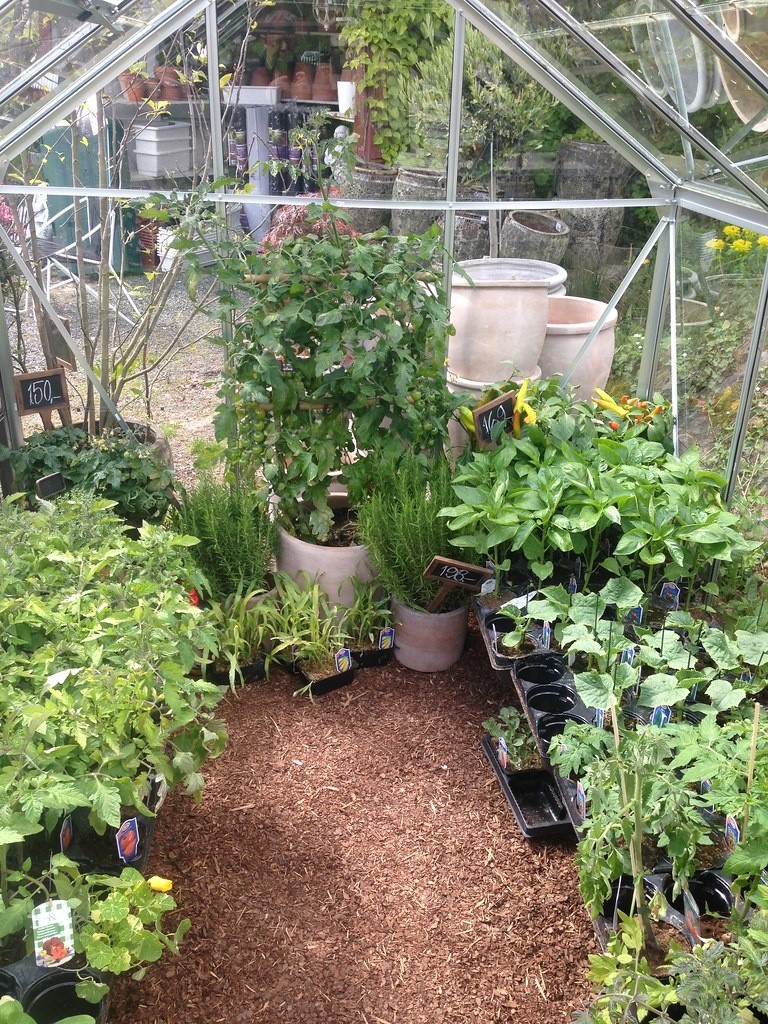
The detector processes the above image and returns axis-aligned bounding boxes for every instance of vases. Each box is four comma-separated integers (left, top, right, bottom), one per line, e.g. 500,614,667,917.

392,167,448,238
118,62,337,102
446,280,551,382
497,209,571,265
340,162,397,233
538,295,618,417
132,136,192,153
133,147,192,177
649,266,763,338
133,120,192,138
438,212,490,264
557,137,628,299
436,372,768,1024
443,366,542,464
416,258,567,297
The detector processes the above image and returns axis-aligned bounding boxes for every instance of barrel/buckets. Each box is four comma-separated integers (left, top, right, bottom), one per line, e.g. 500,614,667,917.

351,137,627,476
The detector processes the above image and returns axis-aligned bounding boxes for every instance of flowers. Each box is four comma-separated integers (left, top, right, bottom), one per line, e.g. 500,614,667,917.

705,226,768,279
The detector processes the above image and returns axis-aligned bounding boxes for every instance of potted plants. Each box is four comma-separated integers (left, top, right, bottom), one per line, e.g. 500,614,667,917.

0,0,292,541
271,594,357,694
246,569,326,675
135,110,455,625
337,575,401,669
354,446,484,672
166,471,278,611
0,662,230,1024
201,578,268,698
